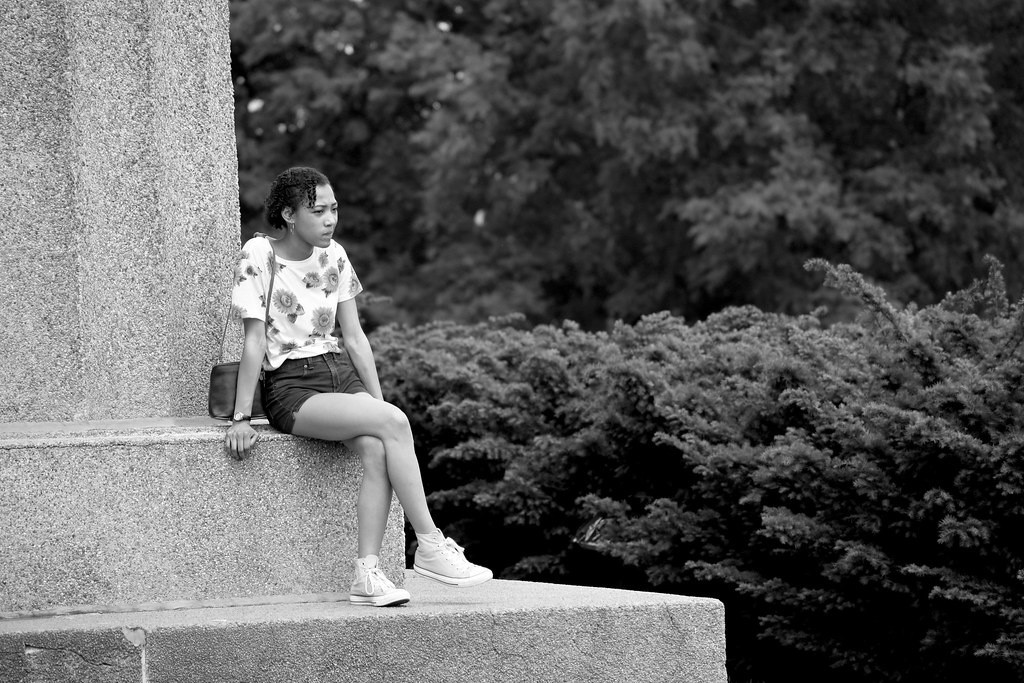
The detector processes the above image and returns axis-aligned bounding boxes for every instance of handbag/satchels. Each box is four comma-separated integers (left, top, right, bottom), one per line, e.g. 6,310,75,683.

207,362,268,421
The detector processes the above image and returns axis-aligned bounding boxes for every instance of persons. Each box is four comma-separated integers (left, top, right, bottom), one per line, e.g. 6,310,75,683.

224,168,493,608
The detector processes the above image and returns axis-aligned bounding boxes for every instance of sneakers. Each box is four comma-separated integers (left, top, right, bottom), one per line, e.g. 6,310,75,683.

411,527,495,590
347,554,411,608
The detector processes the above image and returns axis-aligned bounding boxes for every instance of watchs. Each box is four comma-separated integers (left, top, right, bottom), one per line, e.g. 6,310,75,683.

233,411,252,422
